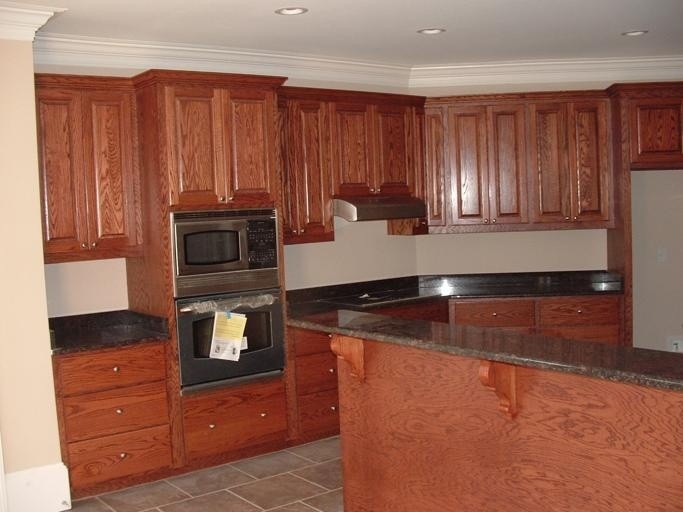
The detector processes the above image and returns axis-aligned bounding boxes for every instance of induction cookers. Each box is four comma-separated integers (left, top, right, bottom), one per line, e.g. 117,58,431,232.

319,287,440,307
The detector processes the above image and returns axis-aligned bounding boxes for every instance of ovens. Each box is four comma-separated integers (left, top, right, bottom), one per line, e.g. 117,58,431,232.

170,299,287,387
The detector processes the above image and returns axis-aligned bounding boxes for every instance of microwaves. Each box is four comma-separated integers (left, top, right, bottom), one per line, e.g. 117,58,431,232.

170,210,280,295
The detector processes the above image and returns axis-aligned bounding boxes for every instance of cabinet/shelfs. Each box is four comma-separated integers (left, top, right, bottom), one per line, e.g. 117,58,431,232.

529,89,617,231
287,300,447,446
448,293,628,346
446,94,529,233
425,97,447,233
51,342,176,501
609,82,682,171
332,90,426,196
165,372,289,476
34,72,144,264
131,69,290,210
276,87,336,246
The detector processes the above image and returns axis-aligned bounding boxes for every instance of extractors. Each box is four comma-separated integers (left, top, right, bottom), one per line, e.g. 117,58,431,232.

332,193,427,223
